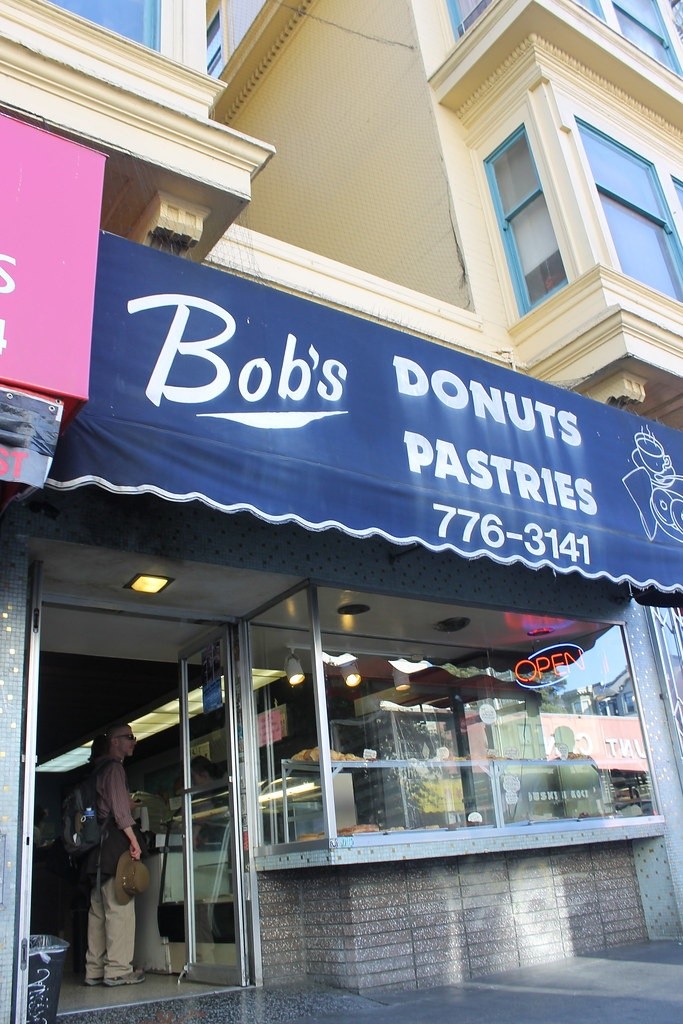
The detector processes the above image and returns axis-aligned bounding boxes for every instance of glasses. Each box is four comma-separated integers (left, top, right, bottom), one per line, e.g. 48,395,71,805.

114,734,134,741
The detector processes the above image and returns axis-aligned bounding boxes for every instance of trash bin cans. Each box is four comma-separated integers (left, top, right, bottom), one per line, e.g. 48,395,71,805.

26,935,70,1024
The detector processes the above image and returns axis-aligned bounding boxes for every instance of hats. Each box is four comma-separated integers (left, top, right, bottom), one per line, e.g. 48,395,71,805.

115,850,150,905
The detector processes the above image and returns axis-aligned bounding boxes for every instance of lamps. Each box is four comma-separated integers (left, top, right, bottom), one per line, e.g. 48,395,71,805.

284,648,305,685
340,653,362,686
392,657,411,691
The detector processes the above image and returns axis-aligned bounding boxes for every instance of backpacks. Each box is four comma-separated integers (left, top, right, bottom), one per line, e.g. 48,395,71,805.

61,758,124,870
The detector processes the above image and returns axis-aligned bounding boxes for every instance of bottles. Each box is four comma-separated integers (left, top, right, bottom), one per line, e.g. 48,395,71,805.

84,808,94,822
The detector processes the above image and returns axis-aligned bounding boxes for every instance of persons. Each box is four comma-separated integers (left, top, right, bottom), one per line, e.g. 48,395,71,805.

191,755,226,852
71,724,145,987
546,726,602,819
33,806,55,849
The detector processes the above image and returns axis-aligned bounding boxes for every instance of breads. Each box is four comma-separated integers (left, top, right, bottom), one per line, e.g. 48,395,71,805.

296,822,440,841
290,746,365,762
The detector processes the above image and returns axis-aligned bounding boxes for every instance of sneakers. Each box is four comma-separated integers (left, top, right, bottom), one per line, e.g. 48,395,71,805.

84,977,104,985
103,972,145,986
